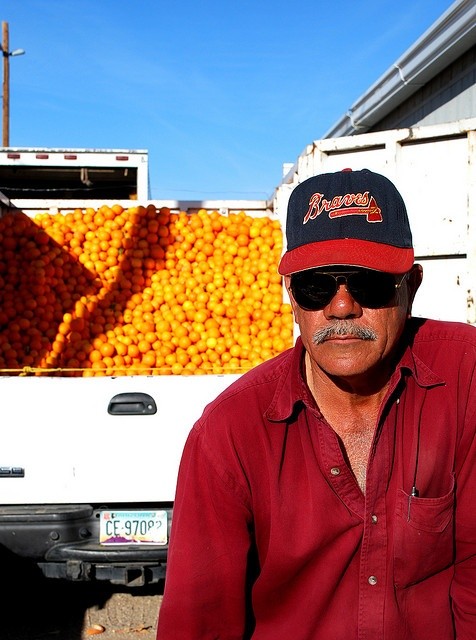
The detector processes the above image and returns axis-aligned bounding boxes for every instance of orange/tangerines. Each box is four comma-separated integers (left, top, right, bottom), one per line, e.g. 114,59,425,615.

0,202,293,374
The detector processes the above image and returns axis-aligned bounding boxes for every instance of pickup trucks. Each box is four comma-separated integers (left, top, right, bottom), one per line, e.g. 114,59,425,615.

0,198,299,590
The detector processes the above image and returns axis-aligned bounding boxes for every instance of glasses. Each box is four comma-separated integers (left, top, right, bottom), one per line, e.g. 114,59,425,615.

290,271,407,310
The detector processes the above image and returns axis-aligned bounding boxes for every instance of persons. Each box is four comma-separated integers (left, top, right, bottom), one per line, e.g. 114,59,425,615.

156,168,475,635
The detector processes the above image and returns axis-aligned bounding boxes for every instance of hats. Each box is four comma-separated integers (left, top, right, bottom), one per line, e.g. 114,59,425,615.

278,168,415,275
